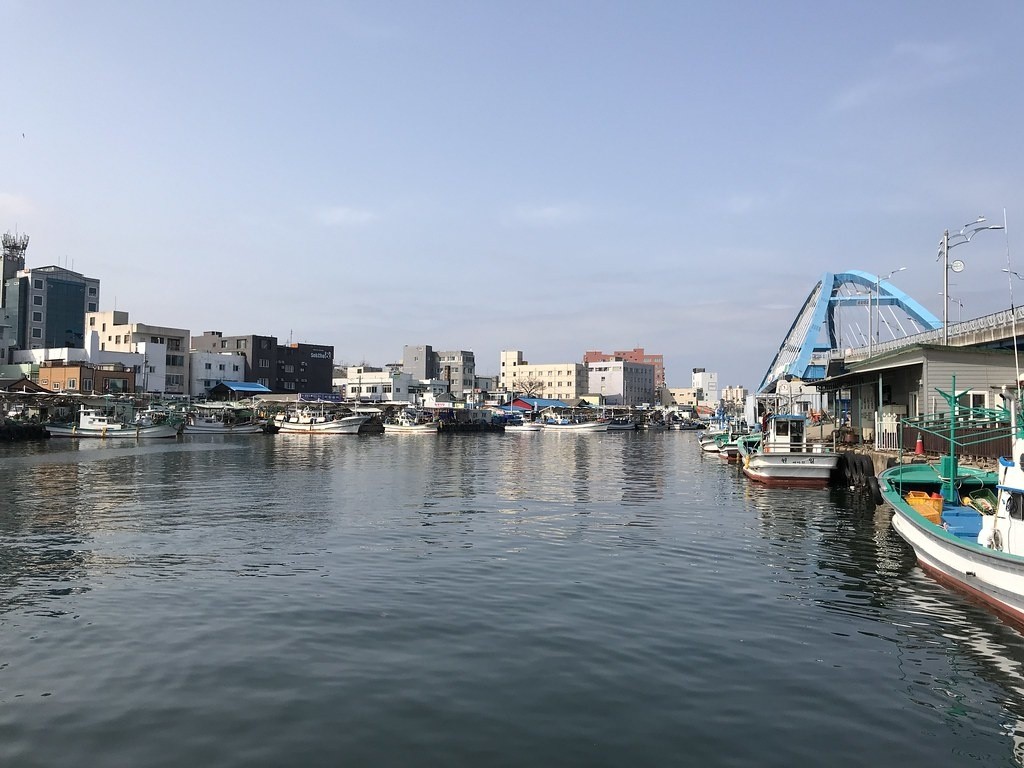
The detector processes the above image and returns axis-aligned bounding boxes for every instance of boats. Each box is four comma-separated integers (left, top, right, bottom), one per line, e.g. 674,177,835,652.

714,416,751,463
382,410,438,434
182,403,262,433
523,421,610,432
645,410,707,429
698,409,732,452
504,423,546,432
878,375,1024,627
45,404,184,437
737,411,841,486
608,419,635,430
274,399,371,434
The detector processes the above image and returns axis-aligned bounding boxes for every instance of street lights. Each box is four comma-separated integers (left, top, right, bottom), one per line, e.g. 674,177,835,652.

938,292,960,322
936,217,1004,346
907,317,926,331
876,267,907,343
1001,268,1024,411
833,288,872,358
881,320,898,339
